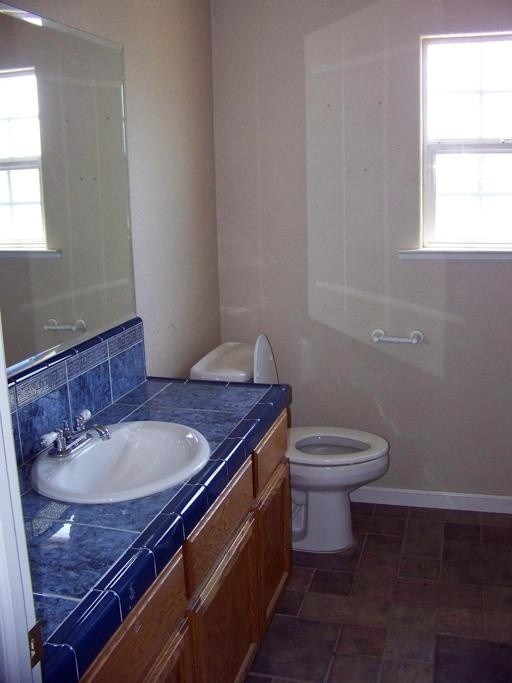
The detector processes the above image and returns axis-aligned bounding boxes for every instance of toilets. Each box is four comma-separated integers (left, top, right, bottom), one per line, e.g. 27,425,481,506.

188,331,391,557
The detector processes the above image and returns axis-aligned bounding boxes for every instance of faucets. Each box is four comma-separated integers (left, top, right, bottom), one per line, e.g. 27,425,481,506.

69,424,112,443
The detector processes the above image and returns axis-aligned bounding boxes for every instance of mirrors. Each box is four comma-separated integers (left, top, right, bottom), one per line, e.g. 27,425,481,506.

0,0,139,373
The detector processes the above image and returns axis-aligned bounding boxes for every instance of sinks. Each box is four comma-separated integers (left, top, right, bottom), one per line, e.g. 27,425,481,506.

30,419,211,506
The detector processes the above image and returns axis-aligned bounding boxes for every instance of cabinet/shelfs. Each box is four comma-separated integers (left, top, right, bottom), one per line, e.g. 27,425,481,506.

193,414,297,682
84,552,192,679
